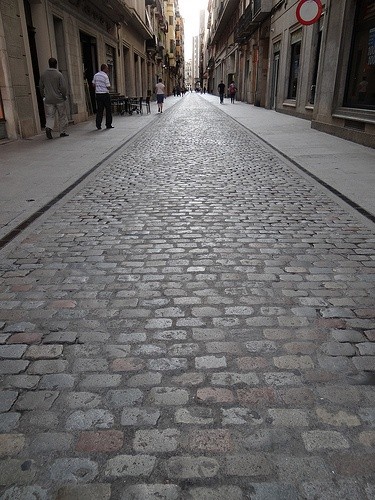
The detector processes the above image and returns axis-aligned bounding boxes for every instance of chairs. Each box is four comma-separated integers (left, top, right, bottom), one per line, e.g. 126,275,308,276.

112,94,151,117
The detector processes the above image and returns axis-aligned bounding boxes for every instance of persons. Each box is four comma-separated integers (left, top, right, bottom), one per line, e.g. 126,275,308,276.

218,81,225,104
176,86,181,97
173,87,176,97
204,87,206,94
38,57,69,139
154,79,165,113
195,87,202,93
91,63,115,129
229,81,238,103
181,85,185,97
207,87,209,93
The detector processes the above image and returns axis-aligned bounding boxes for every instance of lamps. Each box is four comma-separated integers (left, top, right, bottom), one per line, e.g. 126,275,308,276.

116,22,121,28
162,64,166,67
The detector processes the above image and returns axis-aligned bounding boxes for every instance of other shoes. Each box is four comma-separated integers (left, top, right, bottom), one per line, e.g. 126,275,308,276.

158,108,164,113
60,132,70,137
97,126,103,129
107,125,114,128
45,128,53,139
